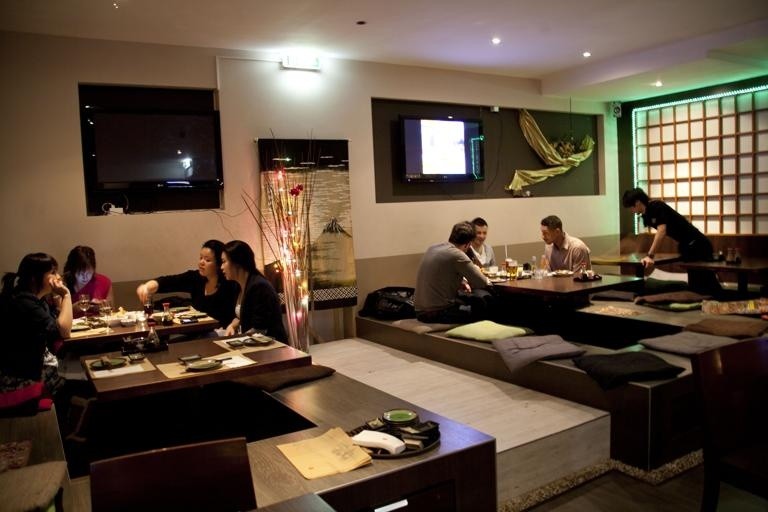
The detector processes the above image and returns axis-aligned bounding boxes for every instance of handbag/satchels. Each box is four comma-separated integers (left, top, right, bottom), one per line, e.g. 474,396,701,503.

359,287,415,320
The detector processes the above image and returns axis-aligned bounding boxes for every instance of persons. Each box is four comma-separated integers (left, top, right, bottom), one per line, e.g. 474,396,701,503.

0,252,89,443
47,246,113,319
136,240,240,328
414,223,488,323
541,216,591,272
465,218,495,267
623,188,712,295
220,240,288,345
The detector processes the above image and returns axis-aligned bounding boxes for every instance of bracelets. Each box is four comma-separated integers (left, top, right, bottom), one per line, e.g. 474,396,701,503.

647,253,654,258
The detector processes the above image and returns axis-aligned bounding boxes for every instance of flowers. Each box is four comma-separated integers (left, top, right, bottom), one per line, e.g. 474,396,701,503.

289,183,304,218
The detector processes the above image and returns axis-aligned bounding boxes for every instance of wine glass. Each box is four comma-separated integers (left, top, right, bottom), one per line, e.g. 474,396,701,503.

79,294,155,333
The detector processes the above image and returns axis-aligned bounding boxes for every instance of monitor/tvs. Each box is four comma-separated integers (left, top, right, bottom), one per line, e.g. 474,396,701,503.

87,106,224,193
398,114,485,185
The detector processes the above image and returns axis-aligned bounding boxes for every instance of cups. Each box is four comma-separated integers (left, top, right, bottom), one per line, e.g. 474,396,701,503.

509,261,518,280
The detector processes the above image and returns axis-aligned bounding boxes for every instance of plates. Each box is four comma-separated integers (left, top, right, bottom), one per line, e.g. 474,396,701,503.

71,306,274,371
348,409,440,459
551,270,574,276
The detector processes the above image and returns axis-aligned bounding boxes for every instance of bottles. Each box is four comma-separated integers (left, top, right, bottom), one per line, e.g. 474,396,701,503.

726,247,736,267
161,302,173,325
530,257,537,276
540,255,548,277
579,263,588,281
734,248,743,267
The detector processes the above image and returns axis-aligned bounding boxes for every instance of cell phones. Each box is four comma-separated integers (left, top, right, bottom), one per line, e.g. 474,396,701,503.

181,317,198,324
89,302,102,305
